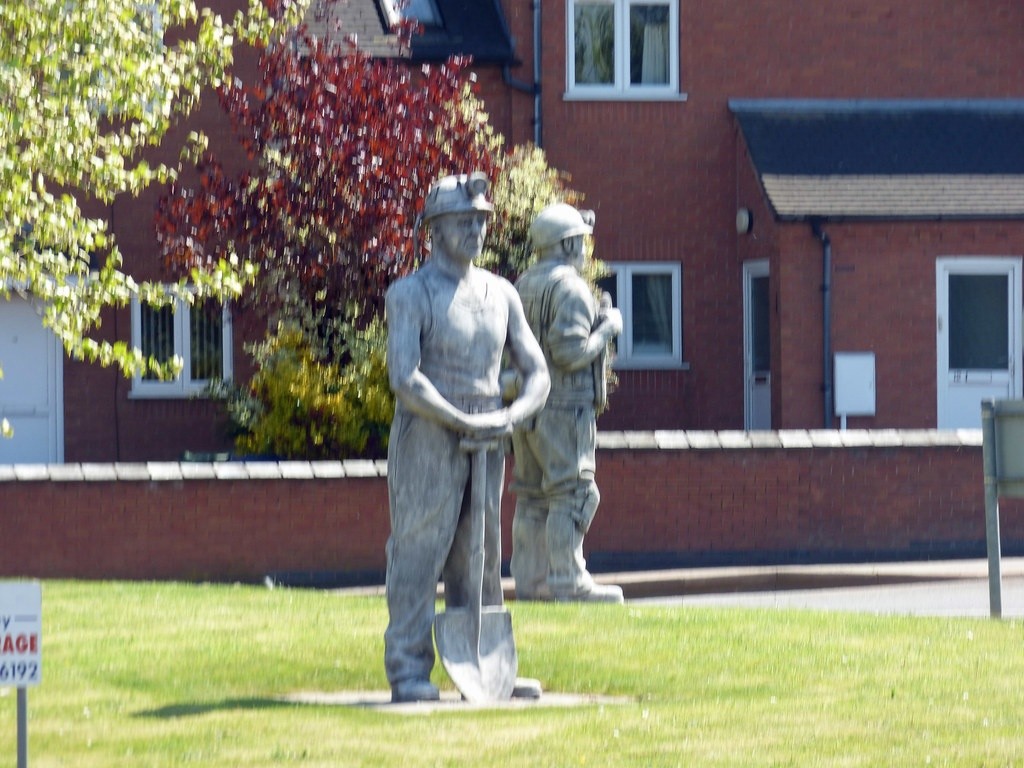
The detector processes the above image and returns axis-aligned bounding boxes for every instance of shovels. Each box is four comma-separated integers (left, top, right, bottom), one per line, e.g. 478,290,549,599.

433,439,518,706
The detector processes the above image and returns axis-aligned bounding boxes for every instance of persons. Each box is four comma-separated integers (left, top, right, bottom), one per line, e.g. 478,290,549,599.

382,172,552,704
509,204,625,602
